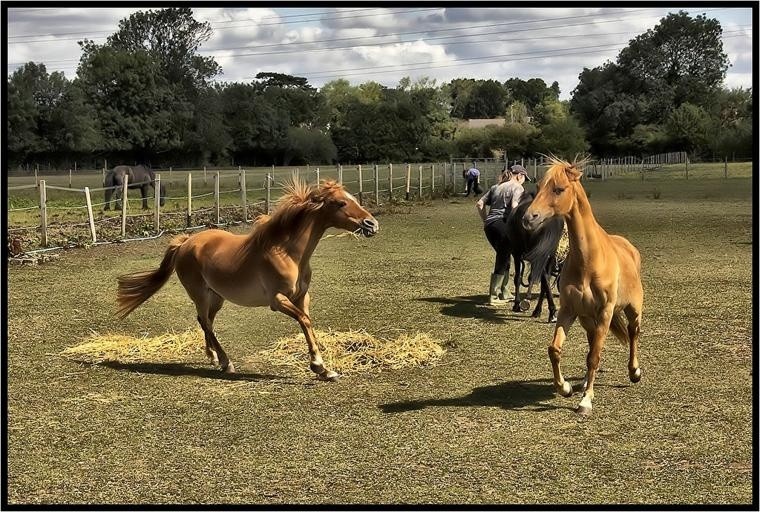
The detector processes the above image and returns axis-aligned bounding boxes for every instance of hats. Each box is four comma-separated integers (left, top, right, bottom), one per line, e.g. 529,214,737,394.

509,165,532,182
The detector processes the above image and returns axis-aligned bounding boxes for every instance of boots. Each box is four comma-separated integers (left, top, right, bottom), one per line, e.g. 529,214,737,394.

499,270,516,301
488,273,509,306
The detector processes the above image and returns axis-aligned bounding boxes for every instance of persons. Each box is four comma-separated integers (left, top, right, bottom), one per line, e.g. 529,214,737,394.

475,163,532,308
463,166,482,197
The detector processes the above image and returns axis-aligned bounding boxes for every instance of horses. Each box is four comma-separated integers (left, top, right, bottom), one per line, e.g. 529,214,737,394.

104,164,166,211
522,150,643,414
501,160,564,323
113,168,379,381
